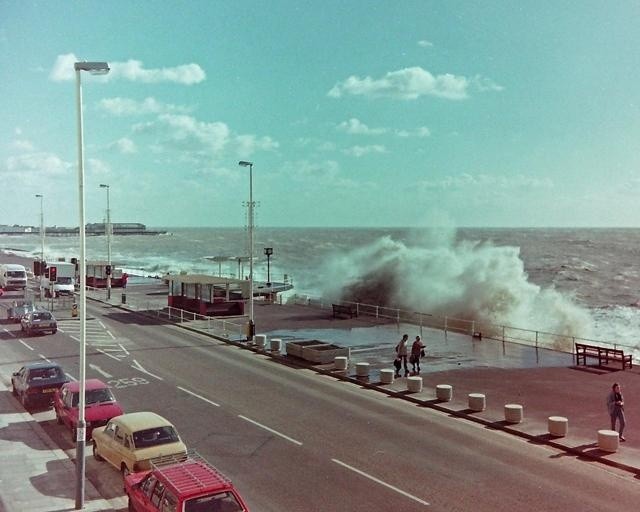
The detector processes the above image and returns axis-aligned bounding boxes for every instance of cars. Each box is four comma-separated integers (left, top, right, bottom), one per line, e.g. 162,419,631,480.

11,363,250,512
21,309,57,335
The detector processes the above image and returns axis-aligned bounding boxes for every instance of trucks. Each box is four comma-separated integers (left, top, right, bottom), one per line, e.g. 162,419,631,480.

0,259,76,298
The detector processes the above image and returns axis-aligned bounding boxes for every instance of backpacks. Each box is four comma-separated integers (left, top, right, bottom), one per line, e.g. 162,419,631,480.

396,340,404,352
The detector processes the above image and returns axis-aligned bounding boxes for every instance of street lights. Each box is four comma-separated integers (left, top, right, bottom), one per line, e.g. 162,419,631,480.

36,61,110,508
238,160,260,322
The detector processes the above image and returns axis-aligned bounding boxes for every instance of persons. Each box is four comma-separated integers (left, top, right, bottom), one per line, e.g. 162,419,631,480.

607,383,626,442
396,334,409,377
410,336,426,375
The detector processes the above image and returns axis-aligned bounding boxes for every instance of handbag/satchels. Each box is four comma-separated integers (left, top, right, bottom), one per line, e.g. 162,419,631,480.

409,355,415,364
393,358,402,369
420,350,425,357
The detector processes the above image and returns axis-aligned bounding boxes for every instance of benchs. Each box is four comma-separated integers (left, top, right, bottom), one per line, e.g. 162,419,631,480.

332,303,357,319
575,342,632,371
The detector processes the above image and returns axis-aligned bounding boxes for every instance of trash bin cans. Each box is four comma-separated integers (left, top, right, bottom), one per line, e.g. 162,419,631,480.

122,293,126,304
72,303,77,317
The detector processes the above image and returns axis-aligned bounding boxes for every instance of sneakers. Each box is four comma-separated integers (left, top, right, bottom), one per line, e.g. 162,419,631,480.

620,436,626,442
